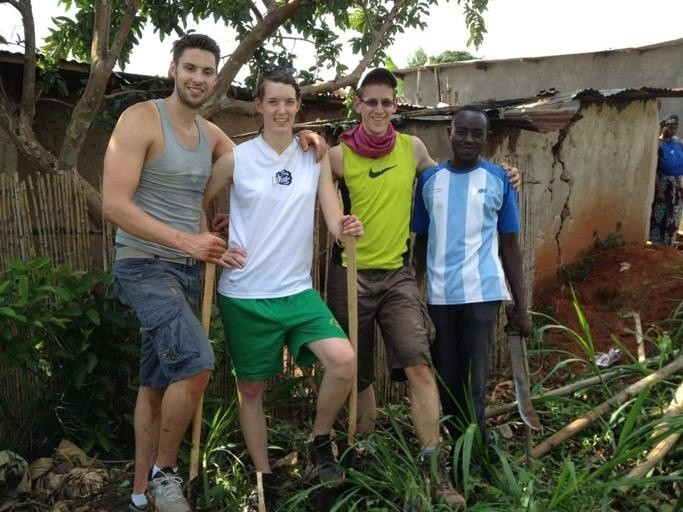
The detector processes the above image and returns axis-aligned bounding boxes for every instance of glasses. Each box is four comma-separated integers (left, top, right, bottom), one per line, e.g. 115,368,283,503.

357,93,395,107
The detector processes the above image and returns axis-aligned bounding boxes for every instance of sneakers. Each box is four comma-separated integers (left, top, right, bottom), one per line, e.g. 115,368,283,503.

129,465,193,512
261,473,280,511
421,458,466,509
352,446,366,468
306,432,346,487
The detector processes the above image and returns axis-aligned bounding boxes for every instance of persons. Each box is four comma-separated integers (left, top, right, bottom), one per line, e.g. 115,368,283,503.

320,66,466,512
649,113,683,247
205,70,357,491
410,108,532,469
100,33,329,512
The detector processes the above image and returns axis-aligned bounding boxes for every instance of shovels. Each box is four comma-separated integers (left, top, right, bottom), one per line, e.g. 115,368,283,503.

310,218,368,512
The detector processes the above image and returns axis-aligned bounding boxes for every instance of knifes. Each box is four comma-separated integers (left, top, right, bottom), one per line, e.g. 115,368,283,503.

505,304,543,430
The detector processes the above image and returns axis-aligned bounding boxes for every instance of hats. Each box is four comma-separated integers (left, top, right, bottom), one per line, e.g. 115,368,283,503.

660,114,678,125
356,67,397,92
